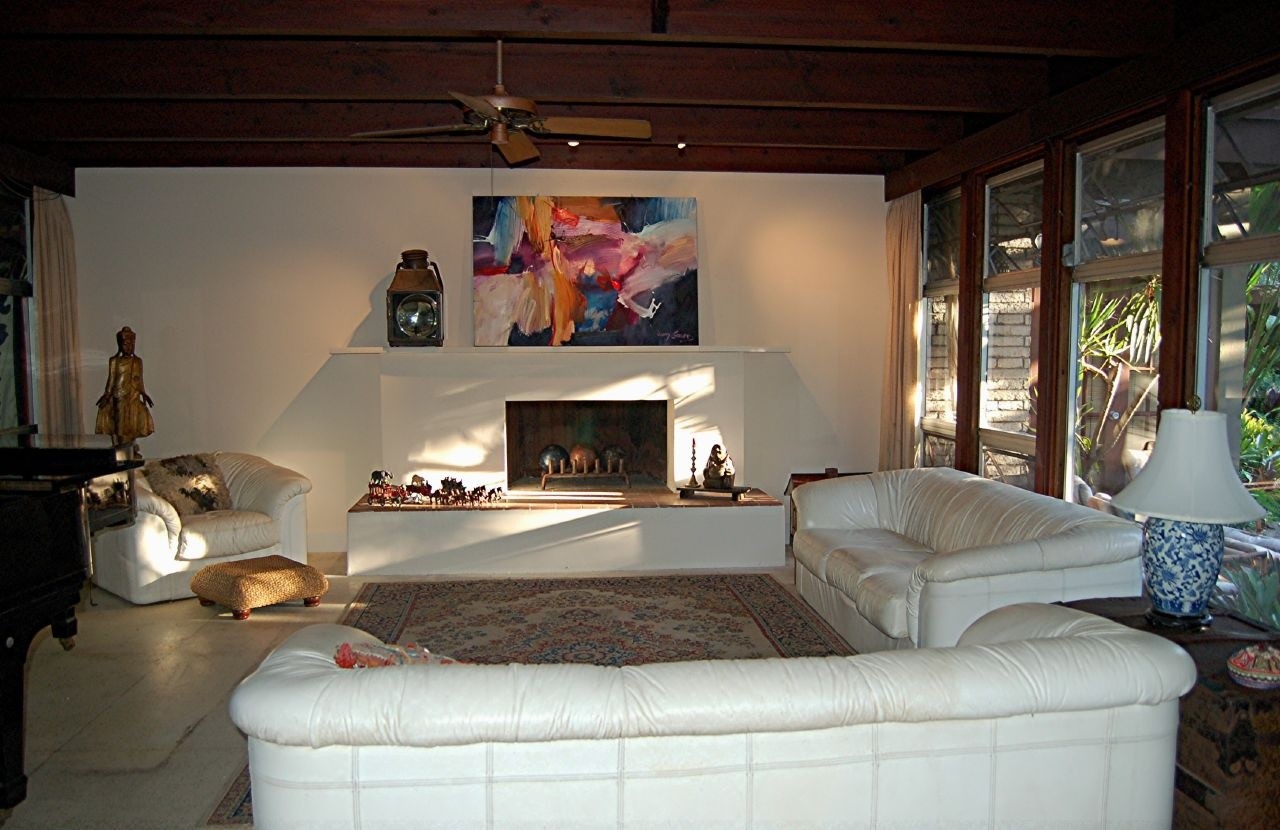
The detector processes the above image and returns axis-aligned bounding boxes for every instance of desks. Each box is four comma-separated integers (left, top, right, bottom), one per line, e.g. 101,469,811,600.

347,494,785,579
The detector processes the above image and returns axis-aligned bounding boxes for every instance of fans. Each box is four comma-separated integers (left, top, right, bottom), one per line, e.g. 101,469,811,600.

351,40,652,166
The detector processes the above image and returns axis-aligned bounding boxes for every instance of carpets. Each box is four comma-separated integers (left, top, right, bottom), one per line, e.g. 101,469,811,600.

196,572,859,829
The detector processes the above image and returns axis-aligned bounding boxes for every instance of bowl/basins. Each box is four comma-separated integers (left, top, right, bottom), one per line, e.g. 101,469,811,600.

1229,654,1280,692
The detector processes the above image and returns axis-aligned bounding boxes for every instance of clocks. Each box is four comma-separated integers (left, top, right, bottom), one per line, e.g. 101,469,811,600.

386,249,444,348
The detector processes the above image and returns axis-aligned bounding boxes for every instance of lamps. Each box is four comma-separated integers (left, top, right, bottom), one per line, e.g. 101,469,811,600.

463,96,539,144
1110,393,1268,627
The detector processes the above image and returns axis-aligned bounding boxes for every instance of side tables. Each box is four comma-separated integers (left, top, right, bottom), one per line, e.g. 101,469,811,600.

1053,597,1280,830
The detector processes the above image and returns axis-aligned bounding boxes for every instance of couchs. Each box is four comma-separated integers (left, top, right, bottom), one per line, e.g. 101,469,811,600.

792,466,1143,654
229,603,1197,830
84,453,311,606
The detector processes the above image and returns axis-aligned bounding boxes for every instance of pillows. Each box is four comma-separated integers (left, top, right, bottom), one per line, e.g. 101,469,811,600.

142,454,232,516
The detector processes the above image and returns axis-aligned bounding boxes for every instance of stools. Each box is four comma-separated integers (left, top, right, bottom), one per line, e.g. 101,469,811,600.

190,554,329,620
